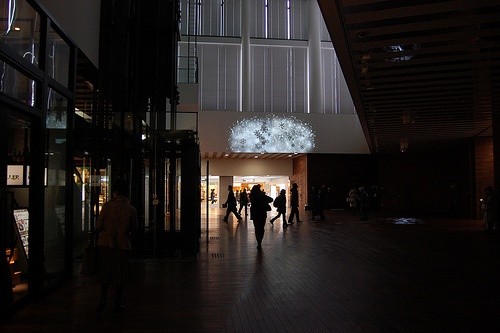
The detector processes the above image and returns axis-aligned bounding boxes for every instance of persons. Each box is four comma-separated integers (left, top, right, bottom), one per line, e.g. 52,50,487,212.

236,189,265,206
310,186,325,222
239,189,248,216
211,189,215,203
349,187,370,220
270,189,289,226
222,185,242,222
481,186,495,231
96,182,137,312
288,183,303,223
250,184,273,255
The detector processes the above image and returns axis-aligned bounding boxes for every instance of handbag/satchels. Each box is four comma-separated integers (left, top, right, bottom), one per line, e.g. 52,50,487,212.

81,231,98,276
